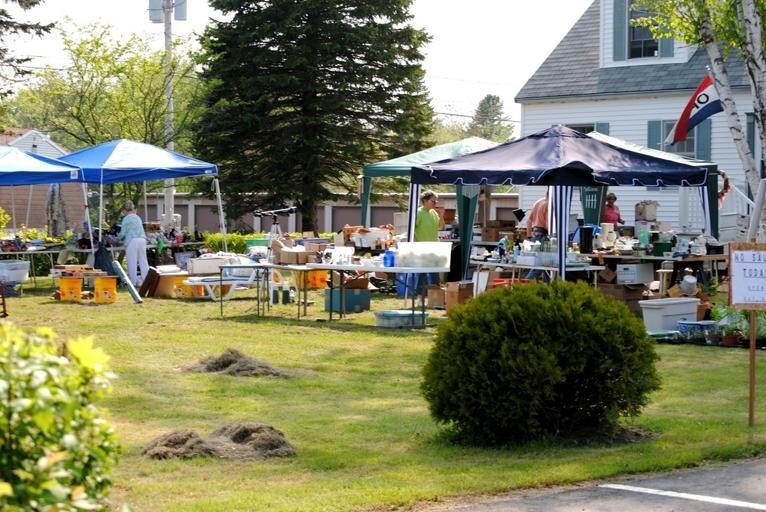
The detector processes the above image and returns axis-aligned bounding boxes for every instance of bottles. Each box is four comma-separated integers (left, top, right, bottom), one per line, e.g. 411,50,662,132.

281,280,289,304
382,250,393,267
513,246,519,262
272,285,278,304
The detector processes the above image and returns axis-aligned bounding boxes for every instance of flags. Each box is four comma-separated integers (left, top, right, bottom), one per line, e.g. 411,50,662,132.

665,73,723,148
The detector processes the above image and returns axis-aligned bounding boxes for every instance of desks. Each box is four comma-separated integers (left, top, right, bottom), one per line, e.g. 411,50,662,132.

219,239,729,327
0,241,209,297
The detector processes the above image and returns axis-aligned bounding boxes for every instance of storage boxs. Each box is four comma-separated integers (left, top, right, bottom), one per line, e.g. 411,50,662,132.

271,219,517,329
542,199,717,344
152,255,229,299
0,259,32,286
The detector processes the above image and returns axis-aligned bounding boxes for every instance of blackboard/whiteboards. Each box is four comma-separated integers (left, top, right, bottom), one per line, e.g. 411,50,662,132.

732,248,765,307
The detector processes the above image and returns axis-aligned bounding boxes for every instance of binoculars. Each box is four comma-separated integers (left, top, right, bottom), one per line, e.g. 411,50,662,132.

253,206,297,217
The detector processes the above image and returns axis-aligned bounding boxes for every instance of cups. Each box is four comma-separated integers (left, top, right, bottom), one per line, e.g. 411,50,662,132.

566,251,576,261
501,256,506,264
507,257,512,263
568,248,572,252
572,242,577,251
491,251,497,259
354,305,360,313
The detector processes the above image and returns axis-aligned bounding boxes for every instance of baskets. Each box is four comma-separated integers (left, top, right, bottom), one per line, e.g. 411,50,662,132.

271,239,326,264
332,271,368,288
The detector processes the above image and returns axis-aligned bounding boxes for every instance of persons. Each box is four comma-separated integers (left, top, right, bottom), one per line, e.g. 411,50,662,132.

414,191,446,310
110,200,150,288
526,190,551,284
602,191,626,238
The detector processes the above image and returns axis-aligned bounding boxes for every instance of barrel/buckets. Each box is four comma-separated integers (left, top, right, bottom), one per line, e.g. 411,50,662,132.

59,276,83,302
492,277,506,286
679,275,699,297
309,270,317,289
318,270,329,289
93,274,117,304
676,319,721,347
291,270,312,290
506,278,529,284
396,273,418,297
418,272,441,297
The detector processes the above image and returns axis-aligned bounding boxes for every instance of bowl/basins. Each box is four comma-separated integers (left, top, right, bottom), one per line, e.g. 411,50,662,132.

631,243,650,250
617,249,634,255
